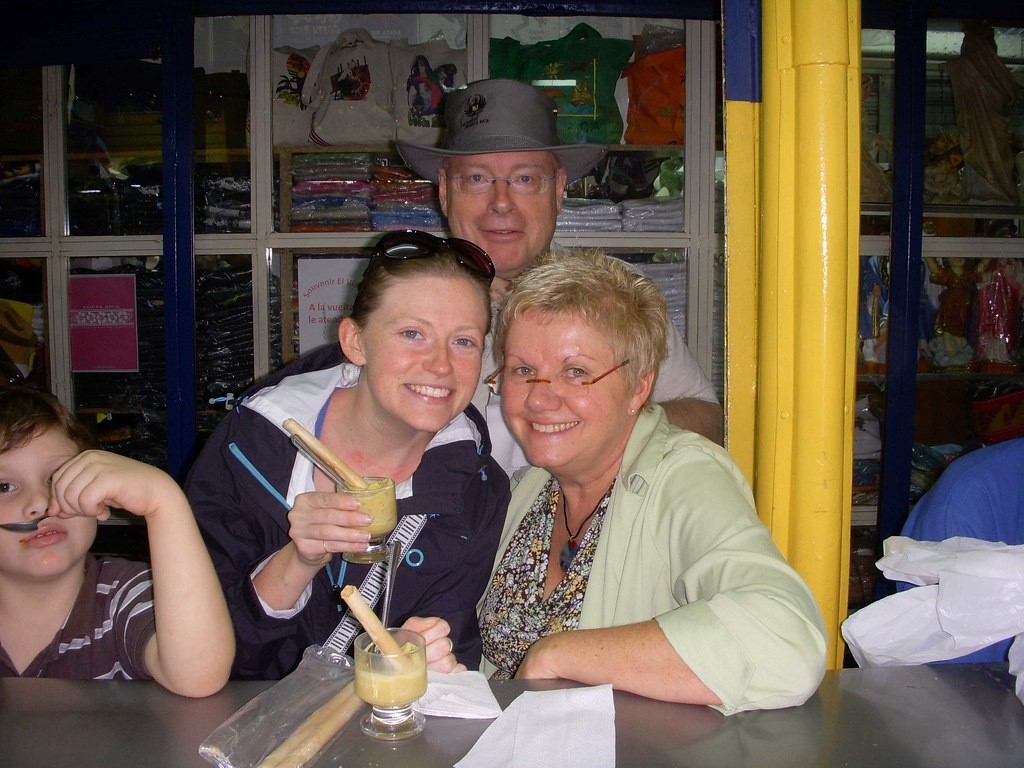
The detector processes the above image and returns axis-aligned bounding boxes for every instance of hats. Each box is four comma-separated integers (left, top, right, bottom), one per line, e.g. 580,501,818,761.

393,78,608,183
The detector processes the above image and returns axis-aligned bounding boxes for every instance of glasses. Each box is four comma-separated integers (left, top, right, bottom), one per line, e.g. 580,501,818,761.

351,229,495,316
443,172,556,196
487,358,631,396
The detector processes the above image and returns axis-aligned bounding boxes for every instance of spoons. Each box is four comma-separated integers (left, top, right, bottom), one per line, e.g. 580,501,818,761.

0,513,49,533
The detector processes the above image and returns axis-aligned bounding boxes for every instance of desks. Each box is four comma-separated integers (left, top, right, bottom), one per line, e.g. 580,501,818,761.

0,661,1024,768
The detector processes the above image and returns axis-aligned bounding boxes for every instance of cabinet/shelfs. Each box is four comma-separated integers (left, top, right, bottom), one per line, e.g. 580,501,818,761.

0,0,1024,666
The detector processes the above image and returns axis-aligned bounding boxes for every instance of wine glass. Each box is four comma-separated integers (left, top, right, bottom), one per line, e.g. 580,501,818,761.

336,476,398,564
354,628,428,741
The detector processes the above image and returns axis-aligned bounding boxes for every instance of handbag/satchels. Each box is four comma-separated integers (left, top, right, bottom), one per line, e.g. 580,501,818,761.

388,30,469,146
620,34,687,143
490,23,635,143
302,28,393,146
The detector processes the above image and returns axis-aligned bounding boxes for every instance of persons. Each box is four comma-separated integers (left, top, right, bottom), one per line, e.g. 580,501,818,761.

0,387,236,698
861,19,1019,204
874,438,1024,665
476,250,829,714
181,248,512,677
397,78,725,477
860,254,1024,366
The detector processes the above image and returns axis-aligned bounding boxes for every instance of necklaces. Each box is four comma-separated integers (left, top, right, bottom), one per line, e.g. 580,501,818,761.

560,494,605,571
